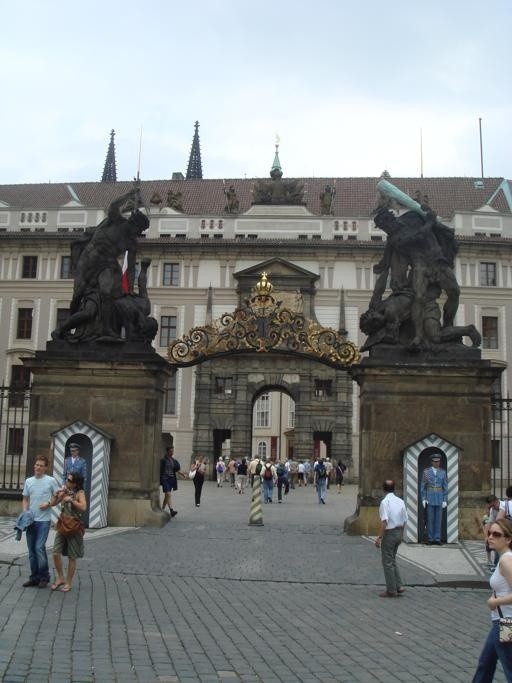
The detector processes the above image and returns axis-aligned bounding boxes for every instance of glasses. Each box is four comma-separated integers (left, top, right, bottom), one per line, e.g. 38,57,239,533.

488,530,502,538
65,477,73,483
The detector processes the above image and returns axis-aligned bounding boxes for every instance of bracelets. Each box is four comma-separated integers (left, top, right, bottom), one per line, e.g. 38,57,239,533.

379,536,383,539
47,498,53,506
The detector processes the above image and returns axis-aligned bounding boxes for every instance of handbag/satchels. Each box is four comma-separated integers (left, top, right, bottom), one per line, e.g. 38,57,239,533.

56,513,84,537
256,464,262,474
189,470,196,479
319,467,325,478
498,617,512,643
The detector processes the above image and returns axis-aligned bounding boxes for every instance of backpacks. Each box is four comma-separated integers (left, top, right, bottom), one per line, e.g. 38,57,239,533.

264,464,272,480
277,462,287,476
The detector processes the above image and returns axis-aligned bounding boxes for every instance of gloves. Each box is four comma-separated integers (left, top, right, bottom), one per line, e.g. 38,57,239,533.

422,500,428,508
442,502,447,508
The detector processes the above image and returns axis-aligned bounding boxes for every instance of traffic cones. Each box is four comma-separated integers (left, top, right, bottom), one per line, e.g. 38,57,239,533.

248,475,264,526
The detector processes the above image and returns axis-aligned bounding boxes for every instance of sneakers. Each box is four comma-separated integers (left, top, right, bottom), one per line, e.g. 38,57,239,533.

171,510,177,516
23,577,48,588
380,586,404,597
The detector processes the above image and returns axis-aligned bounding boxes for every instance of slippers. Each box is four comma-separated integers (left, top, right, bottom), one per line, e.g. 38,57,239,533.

51,580,71,592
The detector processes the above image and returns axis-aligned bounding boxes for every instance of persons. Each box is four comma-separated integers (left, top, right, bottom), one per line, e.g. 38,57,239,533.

23,453,61,590
320,184,336,214
51,473,88,592
421,453,448,546
64,443,89,498
470,518,512,683
359,264,482,348
373,203,460,346
111,257,159,341
52,179,150,339
374,479,409,596
222,184,240,213
481,486,512,572
191,454,207,506
159,445,181,517
217,454,346,503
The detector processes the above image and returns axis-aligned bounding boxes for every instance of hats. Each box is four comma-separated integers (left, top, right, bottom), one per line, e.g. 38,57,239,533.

430,454,441,462
69,443,80,450
